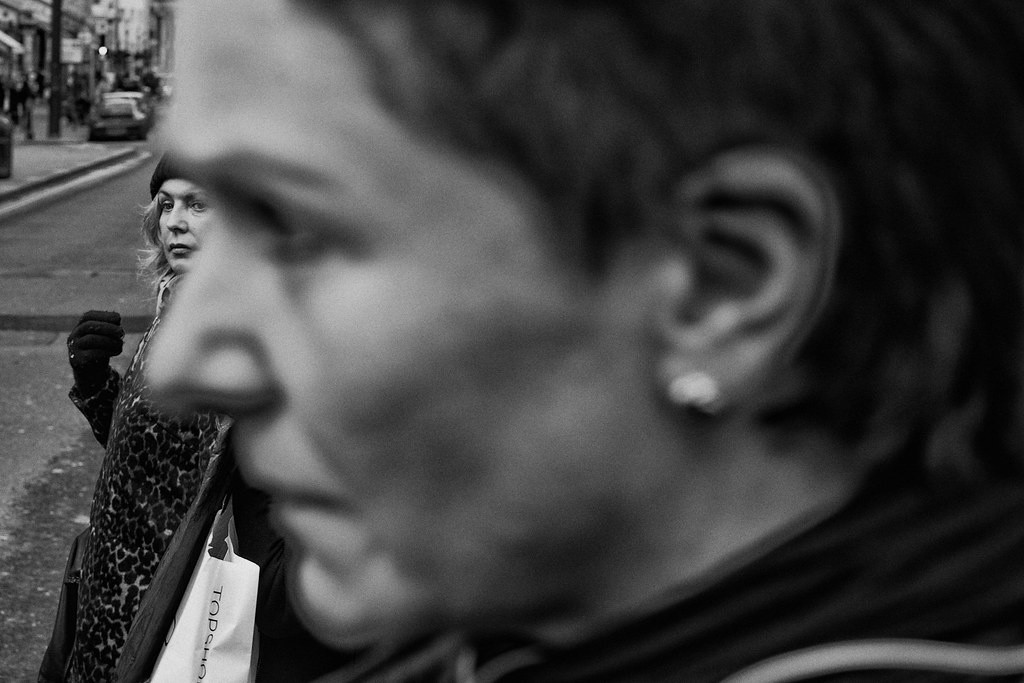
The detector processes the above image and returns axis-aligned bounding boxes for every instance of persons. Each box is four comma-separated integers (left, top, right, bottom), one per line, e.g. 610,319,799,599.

67,152,245,683
0,64,161,125
145,0,1023,683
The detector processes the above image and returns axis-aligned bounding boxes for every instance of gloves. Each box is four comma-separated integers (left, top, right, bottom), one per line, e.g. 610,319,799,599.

67,310,125,399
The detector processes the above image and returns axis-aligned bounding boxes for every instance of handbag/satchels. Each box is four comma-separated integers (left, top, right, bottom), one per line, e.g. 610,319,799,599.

38,525,93,683
145,465,261,683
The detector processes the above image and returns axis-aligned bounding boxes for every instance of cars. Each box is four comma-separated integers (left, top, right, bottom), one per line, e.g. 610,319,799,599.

87,91,149,140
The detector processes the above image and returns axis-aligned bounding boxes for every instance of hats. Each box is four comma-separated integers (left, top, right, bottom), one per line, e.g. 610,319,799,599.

150,150,230,202
